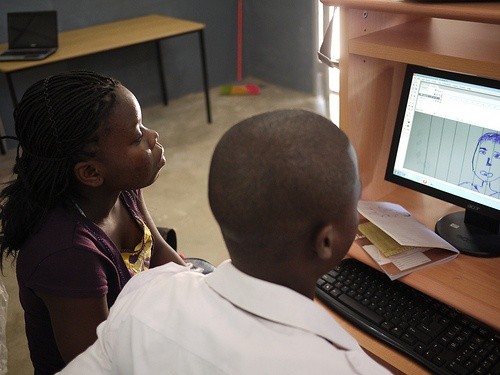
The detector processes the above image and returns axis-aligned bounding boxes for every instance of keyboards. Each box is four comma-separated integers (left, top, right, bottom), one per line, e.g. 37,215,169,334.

316,258,500,375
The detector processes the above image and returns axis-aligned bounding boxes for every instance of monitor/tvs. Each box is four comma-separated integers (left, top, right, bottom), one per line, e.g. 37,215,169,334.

384,62,500,256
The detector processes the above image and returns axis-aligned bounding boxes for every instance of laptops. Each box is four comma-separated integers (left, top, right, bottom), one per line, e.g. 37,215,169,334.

0,10,58,61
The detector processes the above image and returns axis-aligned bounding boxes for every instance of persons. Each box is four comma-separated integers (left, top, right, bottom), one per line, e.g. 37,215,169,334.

0,70,187,375
54,108,393,375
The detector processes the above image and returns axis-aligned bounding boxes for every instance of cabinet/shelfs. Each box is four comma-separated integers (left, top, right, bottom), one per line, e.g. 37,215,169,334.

315,0,500,374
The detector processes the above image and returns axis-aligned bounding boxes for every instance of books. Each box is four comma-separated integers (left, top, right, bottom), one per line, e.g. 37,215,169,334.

355,202,459,281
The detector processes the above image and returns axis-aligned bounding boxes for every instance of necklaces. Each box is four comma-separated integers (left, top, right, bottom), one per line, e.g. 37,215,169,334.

77,192,148,282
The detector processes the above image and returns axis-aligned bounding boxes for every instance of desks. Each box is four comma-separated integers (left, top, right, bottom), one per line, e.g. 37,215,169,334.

0,14,212,125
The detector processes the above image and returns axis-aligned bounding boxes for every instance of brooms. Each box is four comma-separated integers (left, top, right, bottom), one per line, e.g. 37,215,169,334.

218,0,264,97
